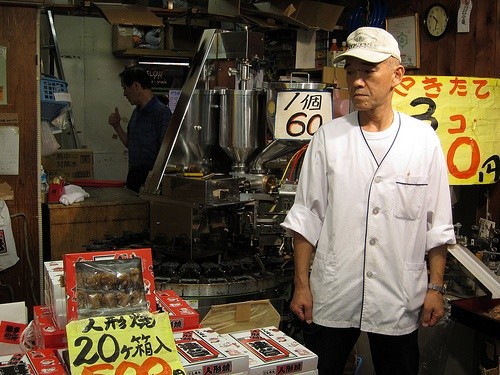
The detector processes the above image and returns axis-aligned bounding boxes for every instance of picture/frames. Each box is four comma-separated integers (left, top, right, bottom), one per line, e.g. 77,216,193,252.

385,12,421,70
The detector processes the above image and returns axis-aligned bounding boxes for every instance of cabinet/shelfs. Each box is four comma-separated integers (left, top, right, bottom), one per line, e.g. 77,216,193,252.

112,23,203,58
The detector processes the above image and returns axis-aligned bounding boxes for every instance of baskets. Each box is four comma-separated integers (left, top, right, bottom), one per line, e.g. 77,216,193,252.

40,78,70,119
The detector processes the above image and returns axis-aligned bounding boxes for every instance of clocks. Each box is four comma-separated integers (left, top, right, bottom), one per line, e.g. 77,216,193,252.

422,4,449,42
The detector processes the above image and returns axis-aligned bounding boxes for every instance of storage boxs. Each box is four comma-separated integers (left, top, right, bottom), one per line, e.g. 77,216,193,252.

39,78,70,118
0,249,319,375
40,148,94,180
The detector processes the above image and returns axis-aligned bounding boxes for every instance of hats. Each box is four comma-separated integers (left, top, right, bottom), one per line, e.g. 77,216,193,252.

332,27,401,64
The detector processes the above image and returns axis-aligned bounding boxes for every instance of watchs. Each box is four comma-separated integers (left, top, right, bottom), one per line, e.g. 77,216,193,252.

428,283,447,294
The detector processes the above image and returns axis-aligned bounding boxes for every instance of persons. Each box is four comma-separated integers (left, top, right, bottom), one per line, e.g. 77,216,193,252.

109,69,174,193
281,27,456,375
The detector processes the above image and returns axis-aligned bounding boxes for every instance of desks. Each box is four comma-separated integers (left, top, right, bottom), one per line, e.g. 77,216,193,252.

47,189,152,258
